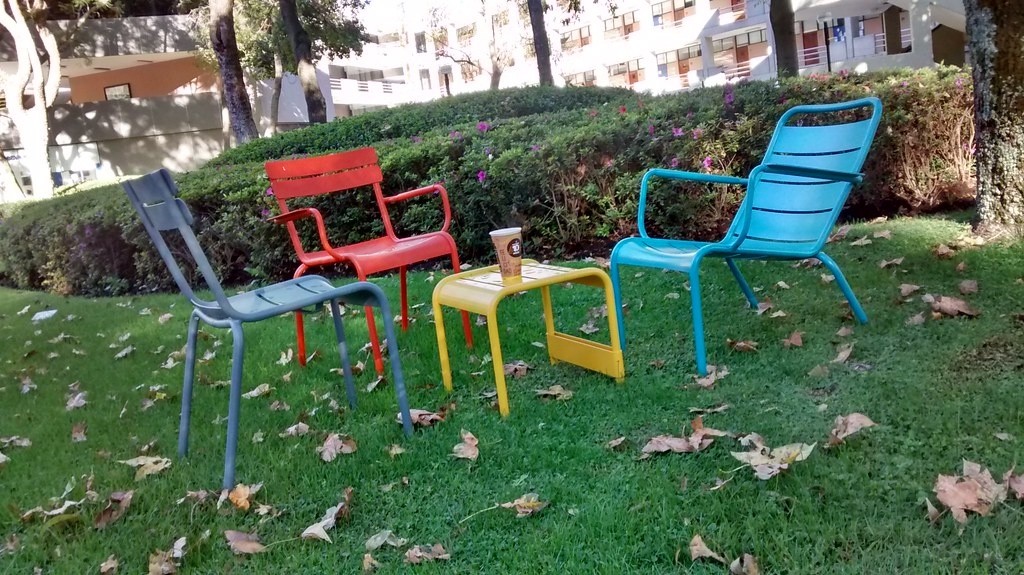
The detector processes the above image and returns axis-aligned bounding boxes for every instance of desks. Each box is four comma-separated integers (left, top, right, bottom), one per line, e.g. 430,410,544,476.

431,257,626,416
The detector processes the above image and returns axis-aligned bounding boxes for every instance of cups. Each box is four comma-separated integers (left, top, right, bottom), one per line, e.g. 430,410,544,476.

488,227,522,285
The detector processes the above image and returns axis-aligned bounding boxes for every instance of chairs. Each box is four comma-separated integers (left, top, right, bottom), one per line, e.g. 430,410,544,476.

264,146,474,377
611,97,883,377
120,167,416,494
